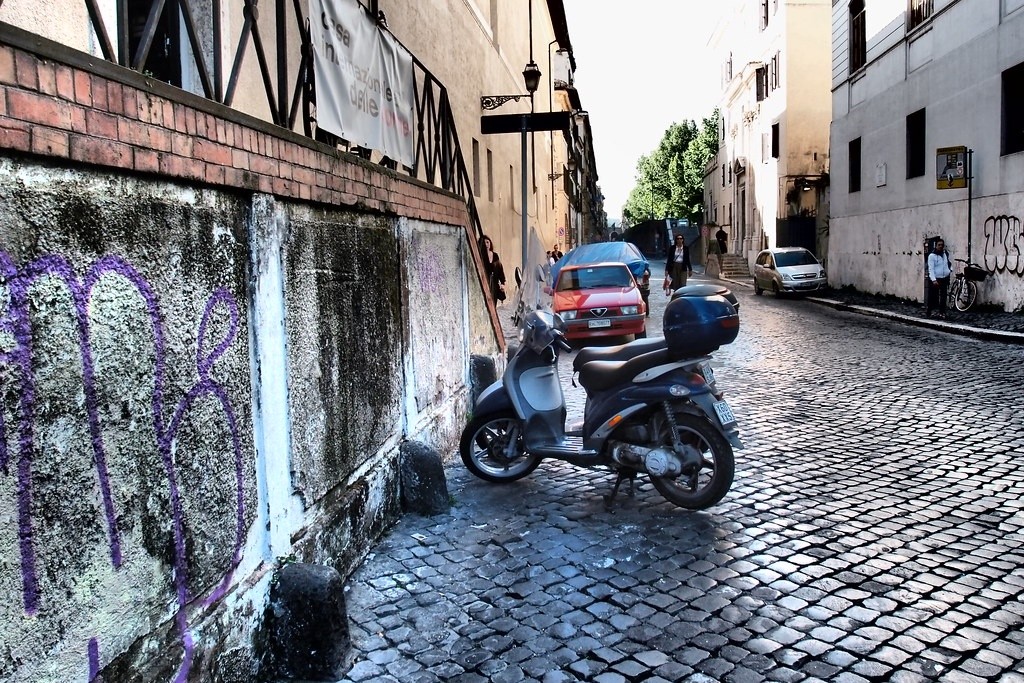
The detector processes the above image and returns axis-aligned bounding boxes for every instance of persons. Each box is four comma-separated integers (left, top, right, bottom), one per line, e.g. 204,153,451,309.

715,225,727,254
478,235,507,308
927,238,953,317
664,234,693,291
544,244,563,286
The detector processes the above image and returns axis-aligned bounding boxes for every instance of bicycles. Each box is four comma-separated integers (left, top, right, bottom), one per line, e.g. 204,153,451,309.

947,258,988,312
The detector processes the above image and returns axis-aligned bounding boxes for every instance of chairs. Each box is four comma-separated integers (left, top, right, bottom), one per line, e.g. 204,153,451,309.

601,273,622,286
566,276,585,289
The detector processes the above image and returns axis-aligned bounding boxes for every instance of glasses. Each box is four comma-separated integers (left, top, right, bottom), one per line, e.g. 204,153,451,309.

677,238,682,240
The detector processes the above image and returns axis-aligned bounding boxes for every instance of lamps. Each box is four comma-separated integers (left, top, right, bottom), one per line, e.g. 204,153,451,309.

803,182,811,191
547,155,578,181
480,58,543,111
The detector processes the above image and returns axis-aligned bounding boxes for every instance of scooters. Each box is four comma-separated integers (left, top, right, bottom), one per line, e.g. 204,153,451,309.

460,227,745,512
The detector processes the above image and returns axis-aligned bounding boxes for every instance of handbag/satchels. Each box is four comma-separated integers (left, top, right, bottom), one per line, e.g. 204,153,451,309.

663,276,671,296
668,262,674,274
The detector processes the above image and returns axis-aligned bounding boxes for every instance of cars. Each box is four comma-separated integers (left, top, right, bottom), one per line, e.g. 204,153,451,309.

752,246,829,299
543,261,646,345
565,241,653,317
